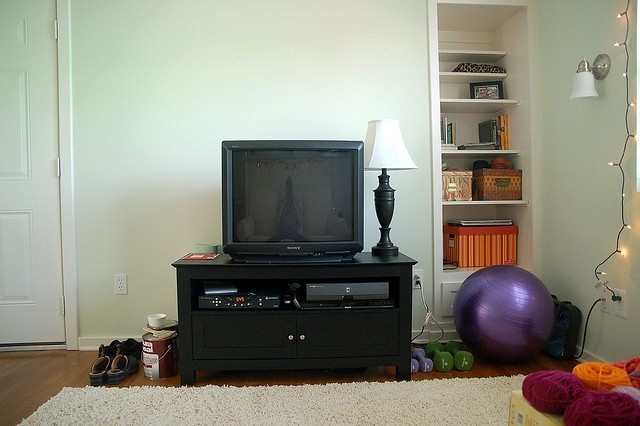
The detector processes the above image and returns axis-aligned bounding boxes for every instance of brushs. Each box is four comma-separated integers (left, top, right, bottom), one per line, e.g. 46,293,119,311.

142,326,175,339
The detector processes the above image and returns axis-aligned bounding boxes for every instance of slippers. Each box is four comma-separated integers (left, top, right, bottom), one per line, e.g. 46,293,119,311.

89,355,115,386
106,354,138,384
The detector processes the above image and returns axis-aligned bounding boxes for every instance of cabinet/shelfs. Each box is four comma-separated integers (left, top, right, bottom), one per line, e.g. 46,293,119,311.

437,2,531,272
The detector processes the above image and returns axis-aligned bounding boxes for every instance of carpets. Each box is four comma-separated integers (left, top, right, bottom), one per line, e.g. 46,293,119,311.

18,373,531,426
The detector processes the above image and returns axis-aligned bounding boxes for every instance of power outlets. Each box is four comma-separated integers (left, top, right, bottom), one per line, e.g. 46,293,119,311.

114,274,127,295
413,269,423,289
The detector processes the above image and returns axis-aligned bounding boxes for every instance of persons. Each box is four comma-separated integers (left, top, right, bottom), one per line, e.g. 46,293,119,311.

475,86,498,99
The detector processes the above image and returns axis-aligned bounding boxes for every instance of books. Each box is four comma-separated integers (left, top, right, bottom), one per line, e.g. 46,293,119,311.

203,283,238,294
182,253,219,260
440,113,510,150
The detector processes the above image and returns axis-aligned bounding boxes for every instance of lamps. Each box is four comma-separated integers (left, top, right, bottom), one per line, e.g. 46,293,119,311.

363,119,419,256
568,55,609,99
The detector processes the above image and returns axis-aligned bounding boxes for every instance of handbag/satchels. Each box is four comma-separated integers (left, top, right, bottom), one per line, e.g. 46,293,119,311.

541,294,581,360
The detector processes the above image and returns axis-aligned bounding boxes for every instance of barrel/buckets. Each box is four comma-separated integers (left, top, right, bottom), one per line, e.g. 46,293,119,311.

139,330,179,380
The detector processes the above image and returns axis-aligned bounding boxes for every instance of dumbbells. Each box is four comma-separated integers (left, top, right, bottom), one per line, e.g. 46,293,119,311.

411,355,419,374
412,348,433,372
426,341,454,371
444,341,474,371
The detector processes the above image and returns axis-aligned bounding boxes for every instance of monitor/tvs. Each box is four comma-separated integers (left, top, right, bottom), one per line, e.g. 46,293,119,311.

223,140,364,264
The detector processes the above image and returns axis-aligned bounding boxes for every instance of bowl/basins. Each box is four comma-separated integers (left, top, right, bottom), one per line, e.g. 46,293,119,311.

147,313,167,327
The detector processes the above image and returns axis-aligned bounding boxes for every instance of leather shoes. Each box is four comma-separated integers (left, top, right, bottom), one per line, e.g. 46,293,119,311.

115,338,143,360
98,340,121,357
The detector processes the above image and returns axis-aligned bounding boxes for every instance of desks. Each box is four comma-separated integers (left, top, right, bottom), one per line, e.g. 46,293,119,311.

170,251,418,387
